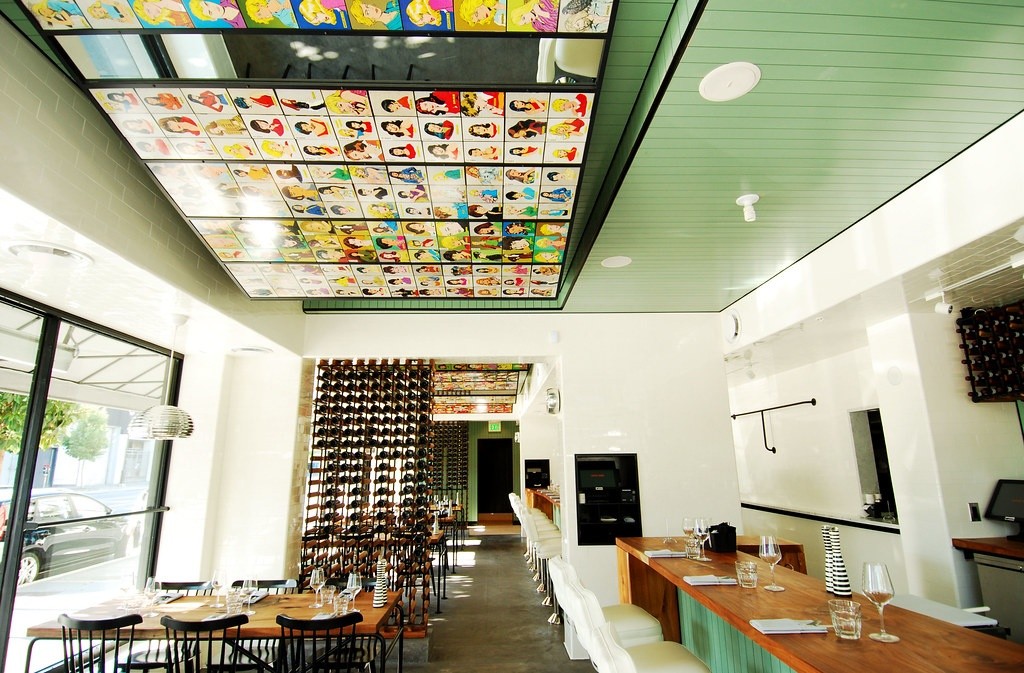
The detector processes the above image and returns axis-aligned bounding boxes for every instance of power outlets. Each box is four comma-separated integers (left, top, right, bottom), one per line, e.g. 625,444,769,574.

969,502,982,522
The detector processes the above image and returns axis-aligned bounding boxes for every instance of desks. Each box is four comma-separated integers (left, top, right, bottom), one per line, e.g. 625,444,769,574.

24,592,405,673
422,515,459,574
426,501,465,549
311,528,451,599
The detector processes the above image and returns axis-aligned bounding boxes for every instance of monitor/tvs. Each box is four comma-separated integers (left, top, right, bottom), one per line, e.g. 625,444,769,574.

984,479,1024,543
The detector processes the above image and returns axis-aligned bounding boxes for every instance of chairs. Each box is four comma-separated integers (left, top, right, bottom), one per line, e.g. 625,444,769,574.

160,614,249,673
324,576,386,661
126,579,216,673
209,580,298,664
307,496,458,612
60,613,143,673
274,612,364,673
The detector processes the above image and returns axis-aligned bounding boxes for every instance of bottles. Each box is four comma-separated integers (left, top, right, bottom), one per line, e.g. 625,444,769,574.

956,306,1024,399
456,492,460,506
433,511,440,535
821,524,852,598
433,422,468,489
448,499,452,516
300,359,435,570
373,559,388,607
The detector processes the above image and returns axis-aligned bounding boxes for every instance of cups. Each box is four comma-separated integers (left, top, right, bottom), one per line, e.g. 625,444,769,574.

684,535,700,559
734,560,758,588
829,599,861,640
320,585,348,617
226,586,243,615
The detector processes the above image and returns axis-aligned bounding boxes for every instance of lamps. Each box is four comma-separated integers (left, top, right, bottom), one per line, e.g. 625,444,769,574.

128,310,196,440
935,291,953,316
545,387,559,414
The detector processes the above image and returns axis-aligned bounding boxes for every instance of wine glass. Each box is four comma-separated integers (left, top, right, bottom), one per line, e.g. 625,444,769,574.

682,516,712,561
759,536,785,592
143,577,162,617
434,494,449,514
862,562,901,642
210,570,259,615
308,569,362,612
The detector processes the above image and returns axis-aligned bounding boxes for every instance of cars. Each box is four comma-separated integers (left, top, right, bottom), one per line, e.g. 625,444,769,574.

0,486,142,587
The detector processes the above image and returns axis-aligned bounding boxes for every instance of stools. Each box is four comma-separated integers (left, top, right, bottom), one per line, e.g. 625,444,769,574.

509,492,711,673
